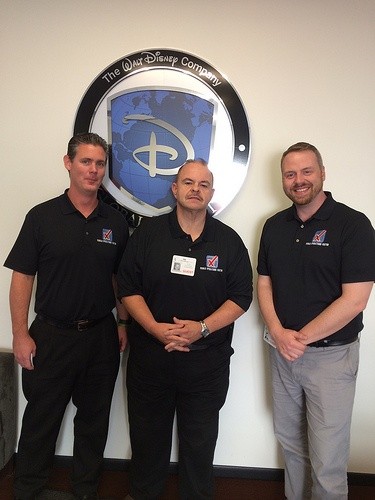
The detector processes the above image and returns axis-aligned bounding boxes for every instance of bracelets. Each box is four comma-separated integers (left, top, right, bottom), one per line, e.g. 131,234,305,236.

117,318,130,324
116,324,129,327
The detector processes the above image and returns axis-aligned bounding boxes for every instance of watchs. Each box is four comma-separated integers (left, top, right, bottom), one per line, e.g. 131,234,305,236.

200,320,210,338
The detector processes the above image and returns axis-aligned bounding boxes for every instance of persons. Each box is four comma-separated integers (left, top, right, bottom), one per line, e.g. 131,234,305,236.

0,133,130,497
257,142,375,500
113,158,254,499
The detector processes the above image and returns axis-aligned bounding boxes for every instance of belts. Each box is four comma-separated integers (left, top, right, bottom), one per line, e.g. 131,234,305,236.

35,313,113,330
308,333,358,347
